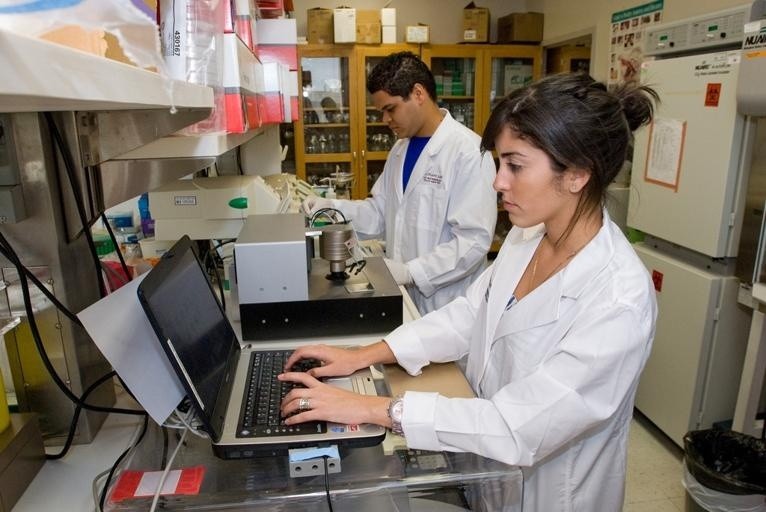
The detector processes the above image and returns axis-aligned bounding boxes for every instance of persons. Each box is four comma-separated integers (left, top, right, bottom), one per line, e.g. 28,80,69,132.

277,70,661,512
299,53,497,377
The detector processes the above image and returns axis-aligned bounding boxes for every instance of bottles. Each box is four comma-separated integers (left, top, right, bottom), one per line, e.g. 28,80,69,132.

306,134,348,154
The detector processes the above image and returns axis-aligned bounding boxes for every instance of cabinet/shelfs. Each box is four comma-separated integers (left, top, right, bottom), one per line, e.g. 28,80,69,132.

294,43,546,200
554,45,591,74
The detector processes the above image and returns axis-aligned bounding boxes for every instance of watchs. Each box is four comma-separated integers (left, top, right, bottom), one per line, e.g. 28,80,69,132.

387,394,406,438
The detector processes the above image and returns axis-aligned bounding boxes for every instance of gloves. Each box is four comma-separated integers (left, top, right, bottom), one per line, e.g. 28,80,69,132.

299,195,335,219
383,258,414,286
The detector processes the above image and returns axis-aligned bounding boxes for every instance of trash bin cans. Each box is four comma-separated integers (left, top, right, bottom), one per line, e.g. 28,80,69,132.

682,428,766,512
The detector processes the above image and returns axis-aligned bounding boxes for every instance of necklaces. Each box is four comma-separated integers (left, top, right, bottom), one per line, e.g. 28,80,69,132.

526,225,602,295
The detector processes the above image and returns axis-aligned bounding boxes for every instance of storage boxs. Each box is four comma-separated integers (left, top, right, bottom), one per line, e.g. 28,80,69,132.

356,9,381,43
222,33,267,133
381,8,397,43
335,8,356,43
256,66,292,122
307,7,333,43
256,19,297,69
405,22,430,43
462,1,491,43
497,12,544,43
231,1,256,51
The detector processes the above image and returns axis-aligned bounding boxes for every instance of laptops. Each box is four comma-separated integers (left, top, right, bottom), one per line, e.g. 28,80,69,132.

137,235,387,460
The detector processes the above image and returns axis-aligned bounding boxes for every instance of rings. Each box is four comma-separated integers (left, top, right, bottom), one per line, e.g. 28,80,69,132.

299,397,309,410
